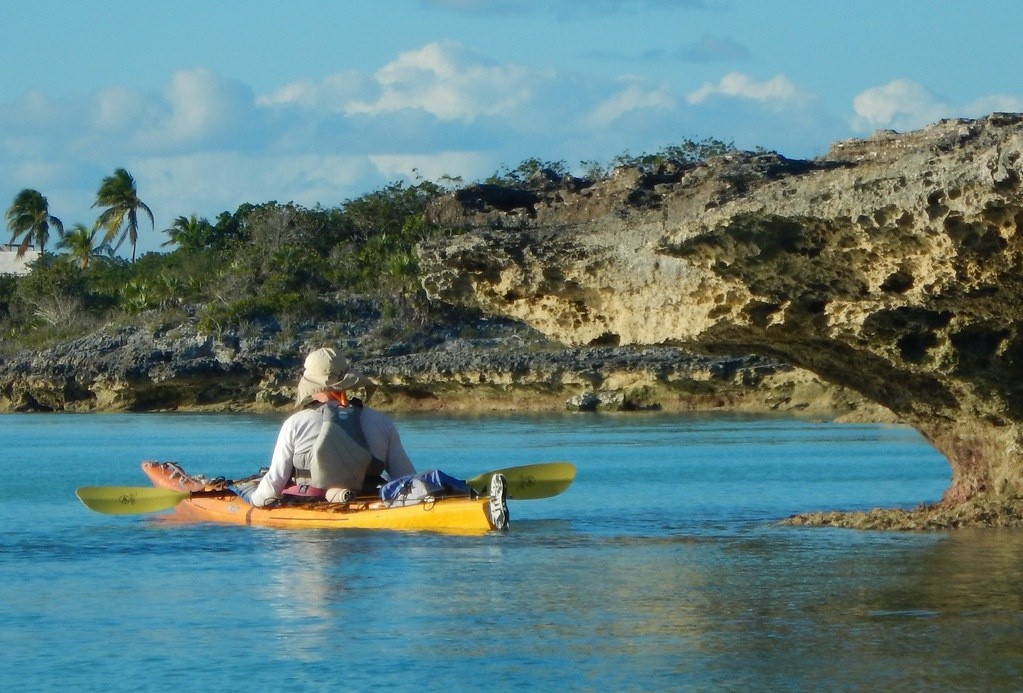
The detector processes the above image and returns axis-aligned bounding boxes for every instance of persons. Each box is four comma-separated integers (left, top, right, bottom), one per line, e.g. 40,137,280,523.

228,348,417,508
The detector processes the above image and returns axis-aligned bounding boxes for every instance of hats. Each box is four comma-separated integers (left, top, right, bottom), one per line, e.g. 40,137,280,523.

293,347,377,409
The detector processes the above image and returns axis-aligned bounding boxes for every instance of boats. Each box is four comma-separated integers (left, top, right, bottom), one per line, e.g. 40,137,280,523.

140,459,512,536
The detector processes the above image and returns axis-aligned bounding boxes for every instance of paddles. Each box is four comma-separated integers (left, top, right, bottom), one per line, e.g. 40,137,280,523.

74,461,577,518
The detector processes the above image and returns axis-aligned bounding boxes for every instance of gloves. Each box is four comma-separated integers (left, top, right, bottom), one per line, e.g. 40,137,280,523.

228,480,258,503
